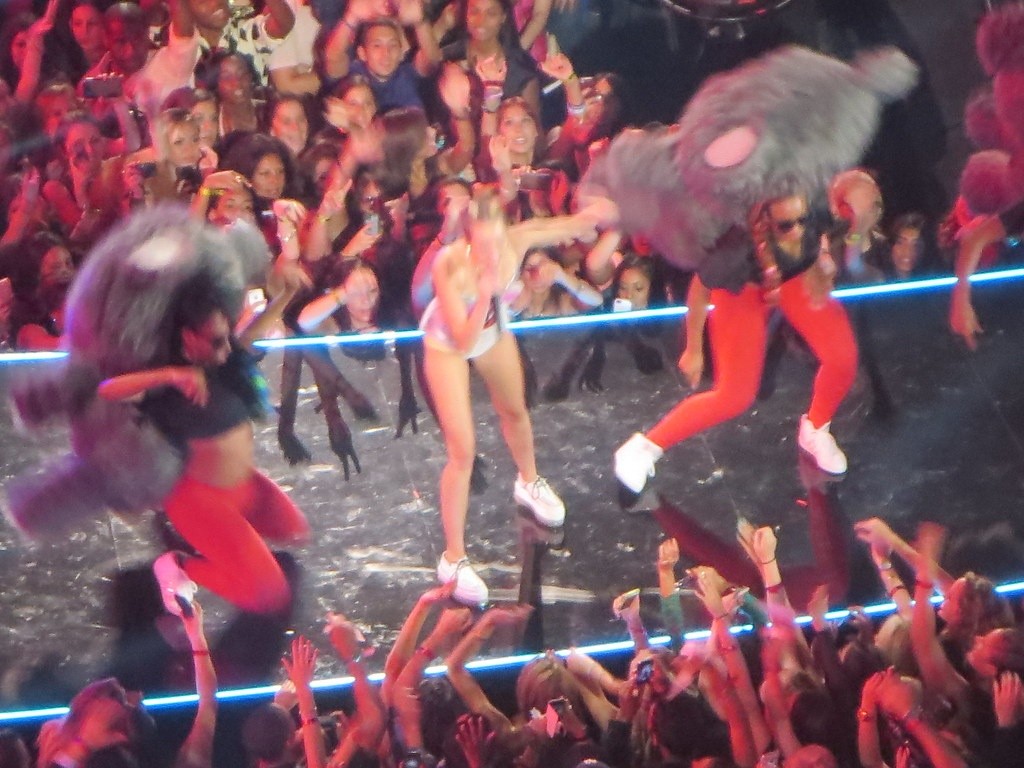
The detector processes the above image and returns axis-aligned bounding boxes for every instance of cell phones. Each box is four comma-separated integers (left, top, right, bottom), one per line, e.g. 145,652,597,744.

735,587,750,599
176,163,198,195
404,751,422,768
548,699,566,721
135,162,155,179
618,588,640,611
637,657,654,686
248,288,266,313
614,299,633,312
83,78,124,98
174,595,194,617
519,173,553,191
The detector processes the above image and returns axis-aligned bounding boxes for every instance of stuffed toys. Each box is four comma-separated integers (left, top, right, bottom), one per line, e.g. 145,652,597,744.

960,2,1023,214
581,43,919,269
3,207,274,550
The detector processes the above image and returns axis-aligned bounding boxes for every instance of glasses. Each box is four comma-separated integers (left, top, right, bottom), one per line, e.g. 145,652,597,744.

191,333,237,351
771,213,808,233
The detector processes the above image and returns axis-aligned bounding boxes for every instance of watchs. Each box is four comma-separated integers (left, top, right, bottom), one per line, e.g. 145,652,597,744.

878,560,894,570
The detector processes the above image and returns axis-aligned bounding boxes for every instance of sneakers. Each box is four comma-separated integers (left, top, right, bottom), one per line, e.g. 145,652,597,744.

436,550,489,606
154,552,198,617
797,413,846,475
513,470,564,527
614,434,663,495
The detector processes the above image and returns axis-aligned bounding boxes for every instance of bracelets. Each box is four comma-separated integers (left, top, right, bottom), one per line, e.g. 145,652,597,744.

70,734,96,757
716,613,728,620
483,81,504,86
852,706,876,721
914,577,934,591
192,650,210,655
481,102,495,114
275,215,290,224
895,707,926,724
761,579,786,595
341,18,355,31
302,718,318,725
331,291,342,307
316,214,331,222
197,156,205,164
627,622,645,634
199,185,211,196
470,626,492,642
415,647,436,661
889,585,906,597
763,558,775,564
575,279,584,296
567,102,585,114
276,228,297,241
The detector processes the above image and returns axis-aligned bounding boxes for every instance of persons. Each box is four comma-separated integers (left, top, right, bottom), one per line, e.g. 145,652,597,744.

0,0,1024,768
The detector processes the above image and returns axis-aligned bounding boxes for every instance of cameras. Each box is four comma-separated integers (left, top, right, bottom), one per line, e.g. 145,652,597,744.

364,213,378,236
